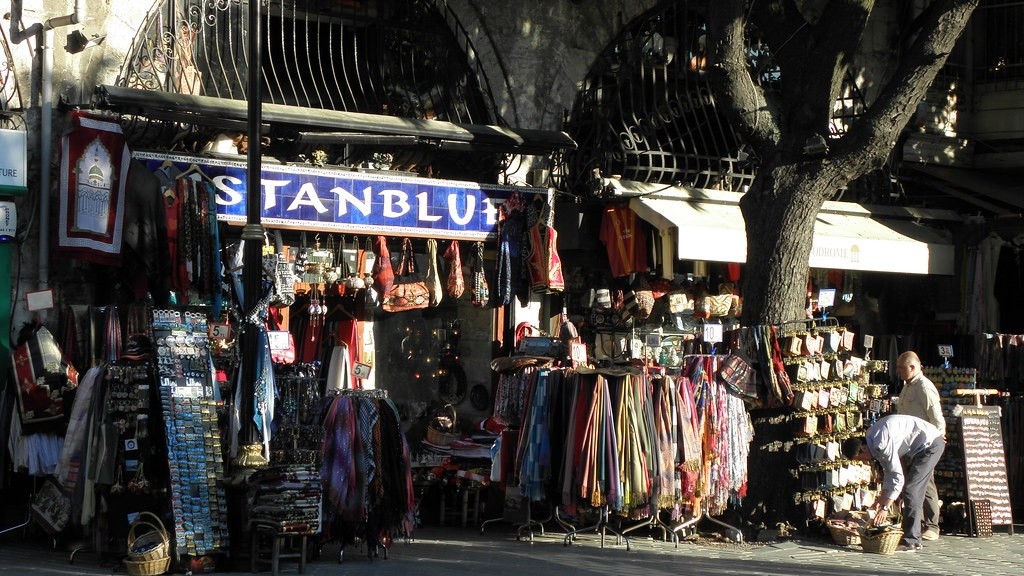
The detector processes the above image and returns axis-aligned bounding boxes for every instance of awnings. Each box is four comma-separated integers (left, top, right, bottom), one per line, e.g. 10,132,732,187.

623,179,963,279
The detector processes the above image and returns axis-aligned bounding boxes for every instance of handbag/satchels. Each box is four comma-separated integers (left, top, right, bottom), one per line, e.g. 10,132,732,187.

265,308,295,365
263,232,295,306
371,235,490,313
516,272,743,369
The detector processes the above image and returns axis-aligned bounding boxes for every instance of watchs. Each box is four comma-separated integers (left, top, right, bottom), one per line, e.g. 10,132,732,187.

881,504,890,511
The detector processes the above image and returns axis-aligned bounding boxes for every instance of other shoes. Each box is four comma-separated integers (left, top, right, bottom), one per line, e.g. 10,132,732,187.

896,541,922,552
922,529,940,540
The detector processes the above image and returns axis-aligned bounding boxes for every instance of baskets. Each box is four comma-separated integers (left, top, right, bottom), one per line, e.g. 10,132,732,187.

122,510,171,576
856,522,904,554
427,403,461,445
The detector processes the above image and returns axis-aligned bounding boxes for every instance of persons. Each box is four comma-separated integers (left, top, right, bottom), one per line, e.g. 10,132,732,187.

893,350,947,541
841,414,945,555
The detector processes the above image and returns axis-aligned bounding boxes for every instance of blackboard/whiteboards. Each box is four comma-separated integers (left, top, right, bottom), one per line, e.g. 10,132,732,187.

960,405,1012,525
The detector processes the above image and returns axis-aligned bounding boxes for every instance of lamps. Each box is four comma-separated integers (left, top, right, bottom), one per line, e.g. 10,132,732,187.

63,22,106,55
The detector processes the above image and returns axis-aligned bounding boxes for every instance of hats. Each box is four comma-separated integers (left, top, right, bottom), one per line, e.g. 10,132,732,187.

119,335,152,359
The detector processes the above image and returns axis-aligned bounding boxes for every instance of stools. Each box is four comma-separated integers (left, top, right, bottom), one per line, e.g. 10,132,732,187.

250,524,307,576
442,484,480,530
